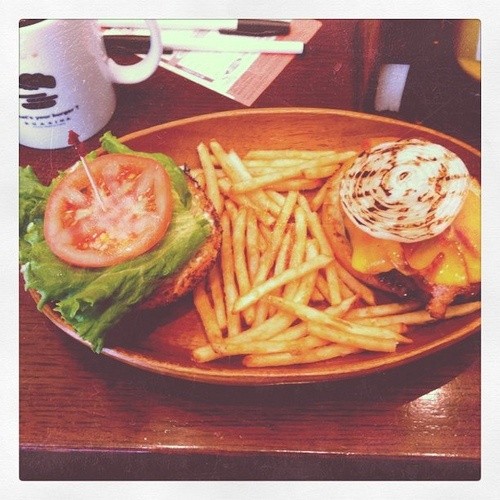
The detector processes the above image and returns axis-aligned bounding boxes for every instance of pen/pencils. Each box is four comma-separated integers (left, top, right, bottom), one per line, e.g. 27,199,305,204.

103,35,307,56
101,19,293,37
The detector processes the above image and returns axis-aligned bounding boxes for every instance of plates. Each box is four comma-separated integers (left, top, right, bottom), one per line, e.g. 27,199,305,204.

19,105,480,384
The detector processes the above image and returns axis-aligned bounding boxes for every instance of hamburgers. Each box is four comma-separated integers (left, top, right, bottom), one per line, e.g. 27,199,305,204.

20,132,223,354
319,130,480,320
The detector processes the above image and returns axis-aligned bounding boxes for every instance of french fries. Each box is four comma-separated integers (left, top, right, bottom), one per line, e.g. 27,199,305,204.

179,136,478,370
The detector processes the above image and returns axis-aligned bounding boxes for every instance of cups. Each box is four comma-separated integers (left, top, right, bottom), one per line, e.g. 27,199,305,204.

19,19,162,150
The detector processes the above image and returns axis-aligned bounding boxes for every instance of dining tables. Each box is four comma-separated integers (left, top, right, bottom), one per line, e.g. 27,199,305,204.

21,19,481,481
